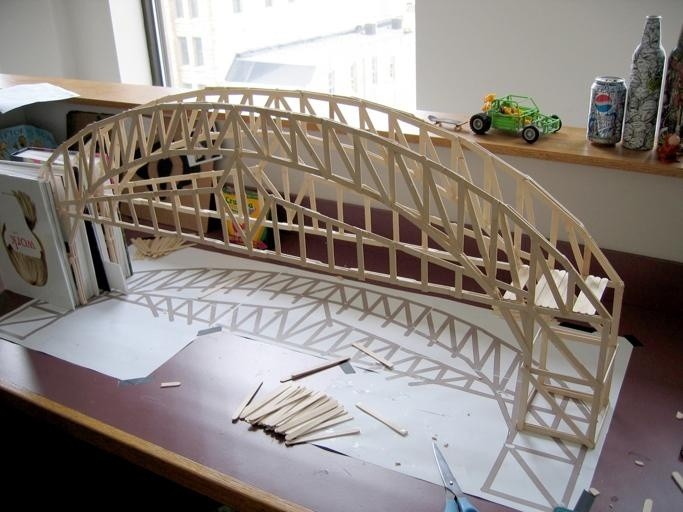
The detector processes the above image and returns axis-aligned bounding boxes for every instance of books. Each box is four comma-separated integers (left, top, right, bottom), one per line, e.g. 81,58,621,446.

221,185,270,249
11,143,135,291
0,156,84,314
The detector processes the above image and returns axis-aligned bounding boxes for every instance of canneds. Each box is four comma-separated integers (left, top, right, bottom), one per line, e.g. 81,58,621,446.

586,76,627,143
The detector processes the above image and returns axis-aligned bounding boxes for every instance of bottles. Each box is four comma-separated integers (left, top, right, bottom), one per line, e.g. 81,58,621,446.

621,14,682,165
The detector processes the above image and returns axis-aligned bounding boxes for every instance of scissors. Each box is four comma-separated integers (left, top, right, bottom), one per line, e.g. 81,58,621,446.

433,440,477,511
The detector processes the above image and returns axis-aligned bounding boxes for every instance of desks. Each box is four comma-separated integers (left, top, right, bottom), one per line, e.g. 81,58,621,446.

0,212,682,512
